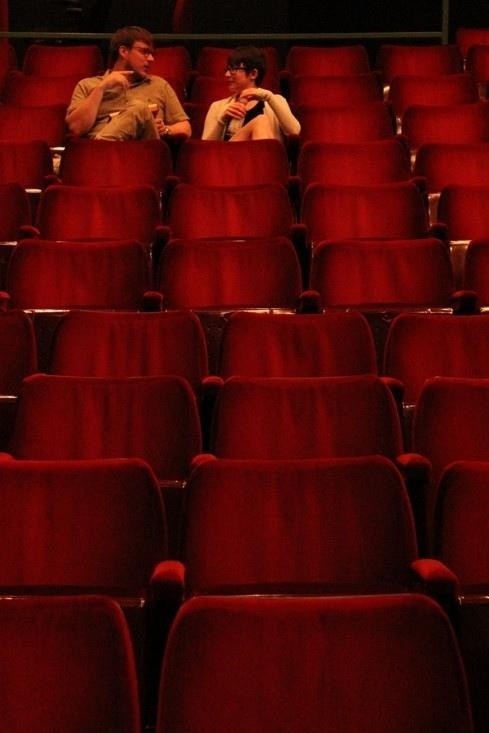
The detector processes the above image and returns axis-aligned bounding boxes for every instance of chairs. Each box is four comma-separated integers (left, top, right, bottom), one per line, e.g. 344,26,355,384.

153,588,476,732
0,594,142,732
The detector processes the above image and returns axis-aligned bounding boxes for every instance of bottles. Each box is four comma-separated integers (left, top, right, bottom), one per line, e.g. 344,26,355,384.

226,97,247,137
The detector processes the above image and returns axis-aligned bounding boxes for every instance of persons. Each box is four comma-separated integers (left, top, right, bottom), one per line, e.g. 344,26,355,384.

66,26,195,147
202,46,303,141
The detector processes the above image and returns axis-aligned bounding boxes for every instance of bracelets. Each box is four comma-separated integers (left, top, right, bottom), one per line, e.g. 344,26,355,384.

217,118,226,126
164,125,169,133
255,87,273,101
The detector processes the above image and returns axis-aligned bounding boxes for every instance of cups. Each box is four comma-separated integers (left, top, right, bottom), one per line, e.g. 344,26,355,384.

149,103,160,119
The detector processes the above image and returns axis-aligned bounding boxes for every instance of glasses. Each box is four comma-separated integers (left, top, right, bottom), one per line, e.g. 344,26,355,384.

132,46,149,56
225,65,245,72
